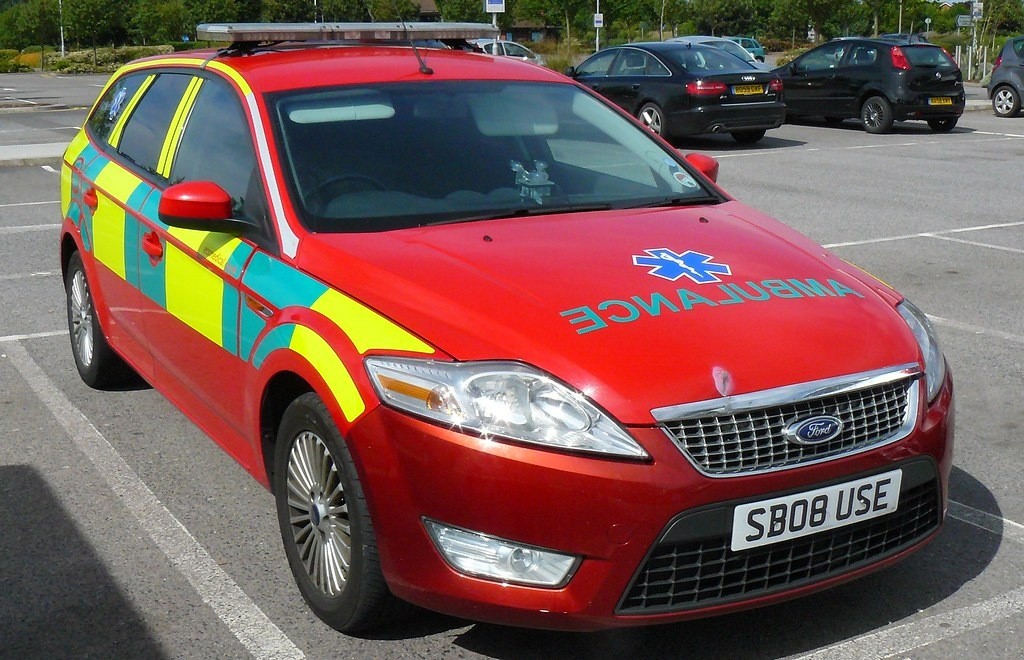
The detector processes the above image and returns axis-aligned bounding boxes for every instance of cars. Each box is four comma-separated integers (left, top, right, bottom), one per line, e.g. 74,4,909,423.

57,21,955,636
723,33,767,65
826,36,875,63
630,32,777,76
882,32,929,46
563,40,788,148
464,37,548,66
986,36,1024,118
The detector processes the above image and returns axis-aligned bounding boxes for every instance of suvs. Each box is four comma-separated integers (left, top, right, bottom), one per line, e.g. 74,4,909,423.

767,35,966,135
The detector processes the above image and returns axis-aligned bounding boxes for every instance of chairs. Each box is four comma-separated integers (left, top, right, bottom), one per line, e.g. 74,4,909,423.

621,54,644,75
856,48,876,65
